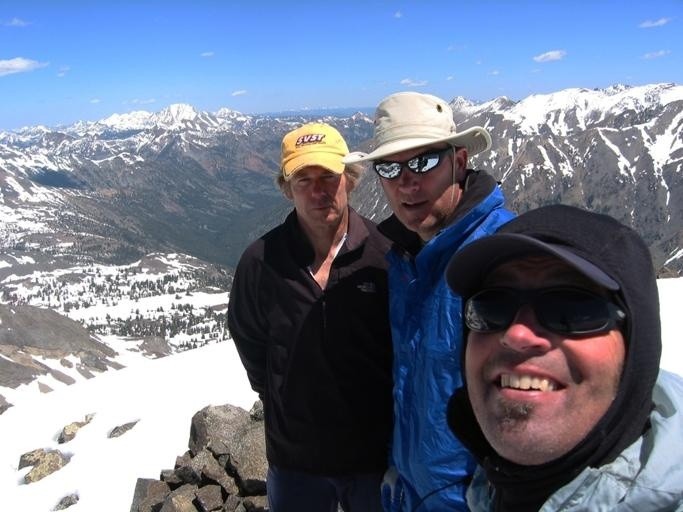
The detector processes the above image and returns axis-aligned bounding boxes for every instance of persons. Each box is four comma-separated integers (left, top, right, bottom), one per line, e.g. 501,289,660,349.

224,122,412,511
437,203,682,512
341,90,521,510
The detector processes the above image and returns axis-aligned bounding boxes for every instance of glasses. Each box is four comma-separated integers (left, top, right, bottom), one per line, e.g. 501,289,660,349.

461,286,624,339
373,149,452,179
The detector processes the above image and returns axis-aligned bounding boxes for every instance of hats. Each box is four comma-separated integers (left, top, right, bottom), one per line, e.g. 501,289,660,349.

281,124,350,181
342,93,492,164
445,232,621,292
